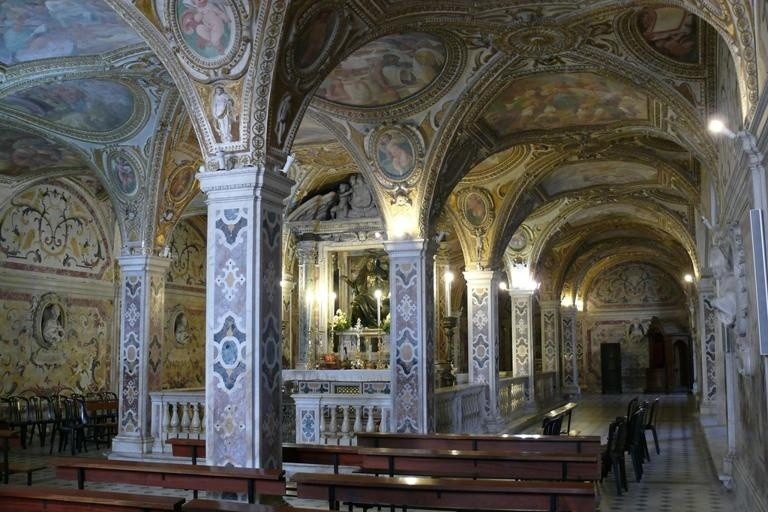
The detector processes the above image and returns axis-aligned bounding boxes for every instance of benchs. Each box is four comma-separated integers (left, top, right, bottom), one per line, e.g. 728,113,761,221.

0,434,604,511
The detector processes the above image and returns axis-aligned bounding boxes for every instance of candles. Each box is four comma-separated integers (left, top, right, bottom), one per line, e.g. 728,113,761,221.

331,303,335,328
377,298,381,328
446,281,453,314
308,302,314,332
357,322,361,351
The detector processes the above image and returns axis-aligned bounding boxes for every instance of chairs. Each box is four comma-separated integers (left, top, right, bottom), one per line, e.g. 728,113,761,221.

0,392,118,455
542,396,659,496
